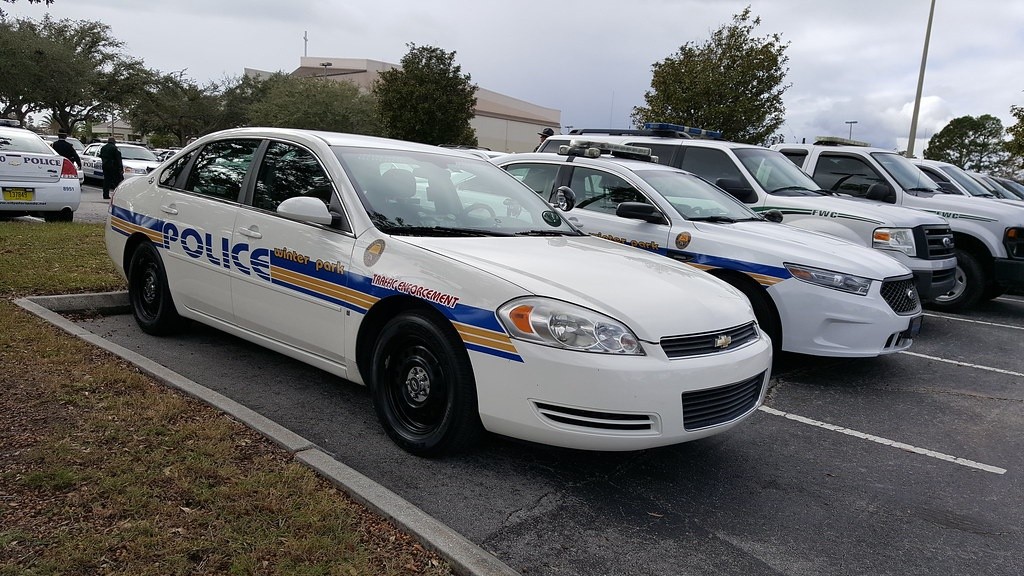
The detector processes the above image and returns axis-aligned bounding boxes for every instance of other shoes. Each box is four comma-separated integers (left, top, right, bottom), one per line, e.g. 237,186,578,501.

103,197,111,199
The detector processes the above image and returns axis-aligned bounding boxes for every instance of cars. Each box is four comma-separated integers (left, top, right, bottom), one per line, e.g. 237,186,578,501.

104,126,774,460
79,142,162,189
416,138,923,378
147,145,182,163
0,118,83,223
36,133,85,186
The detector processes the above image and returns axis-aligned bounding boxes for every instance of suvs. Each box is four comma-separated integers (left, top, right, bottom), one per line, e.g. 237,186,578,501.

521,124,958,311
754,145,1024,312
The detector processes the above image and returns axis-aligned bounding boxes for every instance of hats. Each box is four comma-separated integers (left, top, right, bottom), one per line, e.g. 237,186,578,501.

57,129,68,134
538,128,554,137
107,137,117,143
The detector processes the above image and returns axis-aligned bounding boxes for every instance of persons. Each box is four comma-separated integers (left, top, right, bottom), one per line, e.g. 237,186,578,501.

90,134,101,145
100,138,124,199
533,128,555,153
53,129,82,170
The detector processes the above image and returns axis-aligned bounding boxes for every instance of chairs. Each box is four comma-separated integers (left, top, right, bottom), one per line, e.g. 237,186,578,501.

382,169,428,227
